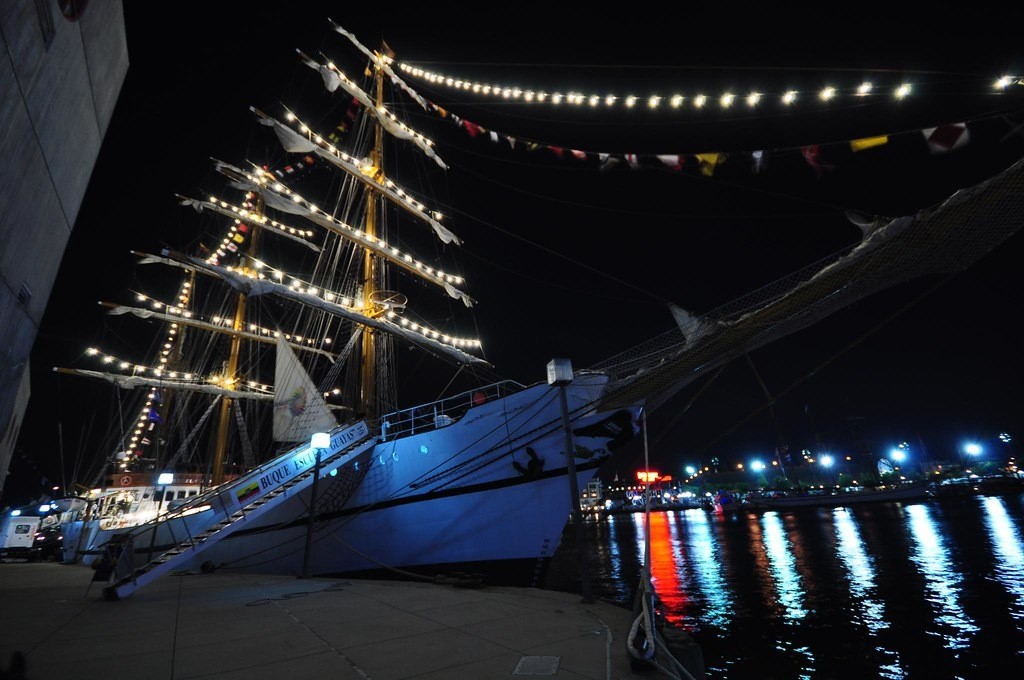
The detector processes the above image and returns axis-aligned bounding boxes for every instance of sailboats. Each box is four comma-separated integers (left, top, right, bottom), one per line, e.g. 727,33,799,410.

56,15,1024,574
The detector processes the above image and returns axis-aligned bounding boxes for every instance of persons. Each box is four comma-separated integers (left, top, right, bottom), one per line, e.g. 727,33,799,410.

432,410,455,429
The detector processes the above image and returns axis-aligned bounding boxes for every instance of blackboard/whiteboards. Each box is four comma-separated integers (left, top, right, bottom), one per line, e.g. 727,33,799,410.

92,534,135,582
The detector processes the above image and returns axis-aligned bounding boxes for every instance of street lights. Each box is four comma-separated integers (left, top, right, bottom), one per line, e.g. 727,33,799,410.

297,431,331,581
545,358,595,602
146,473,174,560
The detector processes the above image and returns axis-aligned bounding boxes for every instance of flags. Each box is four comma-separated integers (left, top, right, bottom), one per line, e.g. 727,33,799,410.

244,194,257,209
276,156,314,177
327,98,363,144
141,438,151,445
150,409,164,425
427,100,724,176
212,224,248,266
751,122,971,177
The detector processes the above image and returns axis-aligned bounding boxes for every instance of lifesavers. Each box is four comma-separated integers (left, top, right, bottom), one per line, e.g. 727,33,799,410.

120,476,133,487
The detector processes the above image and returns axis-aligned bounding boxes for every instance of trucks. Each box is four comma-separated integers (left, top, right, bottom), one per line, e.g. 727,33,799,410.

0,515,41,563
